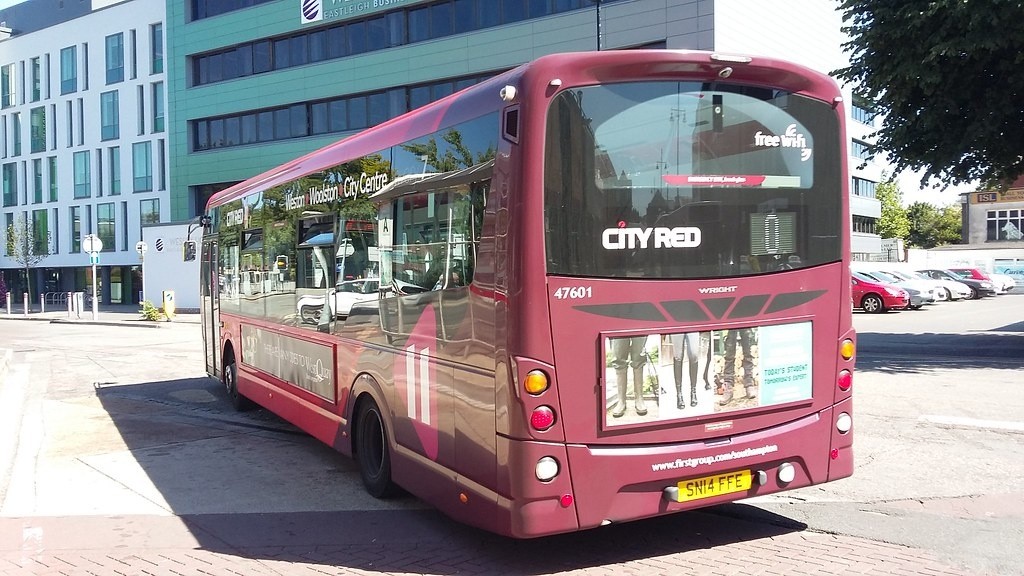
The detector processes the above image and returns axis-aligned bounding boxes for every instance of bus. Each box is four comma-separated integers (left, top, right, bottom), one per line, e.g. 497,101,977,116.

181,50,858,541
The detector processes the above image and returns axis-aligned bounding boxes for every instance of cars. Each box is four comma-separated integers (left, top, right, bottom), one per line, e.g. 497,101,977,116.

291,272,427,326
849,262,1017,314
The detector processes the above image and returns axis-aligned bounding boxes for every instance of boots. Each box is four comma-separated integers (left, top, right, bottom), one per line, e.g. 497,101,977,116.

688,362,698,407
633,368,648,414
612,368,628,416
673,357,685,409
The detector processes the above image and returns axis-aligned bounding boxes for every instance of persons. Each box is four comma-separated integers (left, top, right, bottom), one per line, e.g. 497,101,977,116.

717,327,756,406
611,336,648,418
669,331,701,408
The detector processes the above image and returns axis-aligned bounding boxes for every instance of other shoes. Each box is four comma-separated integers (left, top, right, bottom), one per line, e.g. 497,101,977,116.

720,386,734,405
745,386,756,398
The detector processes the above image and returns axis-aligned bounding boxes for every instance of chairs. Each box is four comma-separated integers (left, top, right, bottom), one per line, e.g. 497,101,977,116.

551,201,758,279
